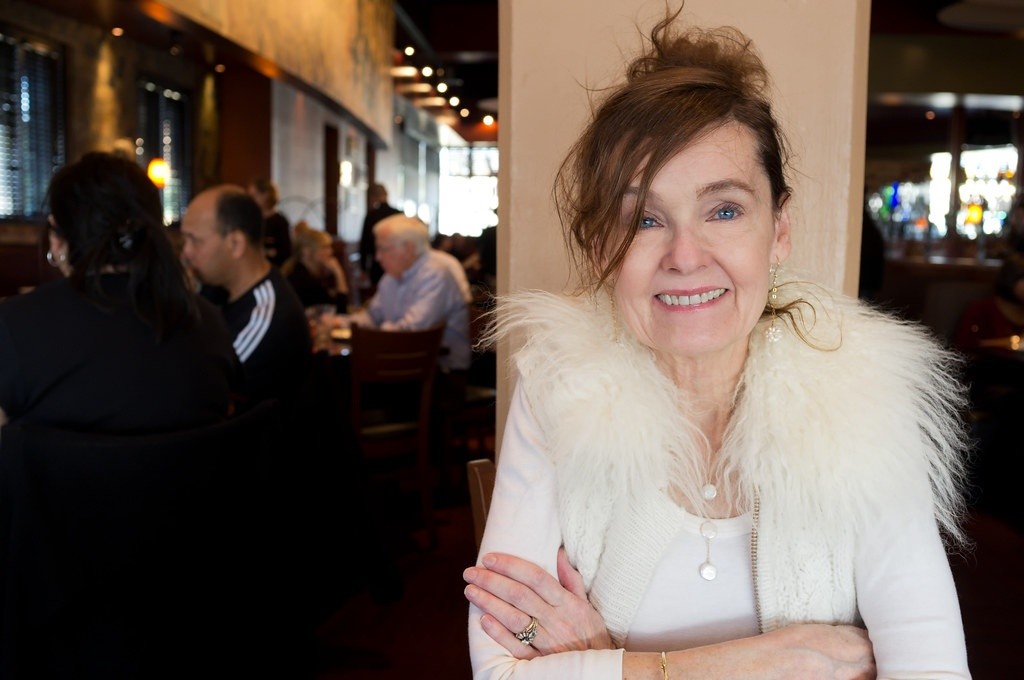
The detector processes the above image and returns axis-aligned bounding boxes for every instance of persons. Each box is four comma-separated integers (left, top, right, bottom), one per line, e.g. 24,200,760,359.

1,152,236,502
463,32,969,680
950,105,1024,539
170,181,498,408
858,209,887,304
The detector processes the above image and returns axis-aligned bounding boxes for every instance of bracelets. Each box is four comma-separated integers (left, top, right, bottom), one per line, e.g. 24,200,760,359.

661,652,669,680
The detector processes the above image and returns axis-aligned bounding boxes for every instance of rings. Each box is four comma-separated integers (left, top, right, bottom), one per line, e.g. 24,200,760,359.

516,616,538,645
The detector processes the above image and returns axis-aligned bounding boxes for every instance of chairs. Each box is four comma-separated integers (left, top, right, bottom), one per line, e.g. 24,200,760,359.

348,326,443,550
